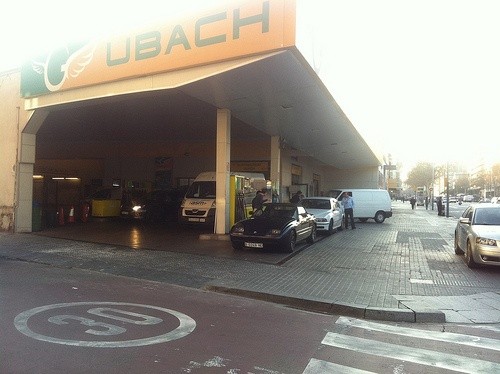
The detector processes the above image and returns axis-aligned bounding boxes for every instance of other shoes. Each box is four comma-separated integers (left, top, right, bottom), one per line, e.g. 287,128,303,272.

352,226,356,230
345,226,348,229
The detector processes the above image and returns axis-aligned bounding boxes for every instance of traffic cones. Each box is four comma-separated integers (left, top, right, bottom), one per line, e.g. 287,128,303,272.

56,208,64,225
67,206,75,223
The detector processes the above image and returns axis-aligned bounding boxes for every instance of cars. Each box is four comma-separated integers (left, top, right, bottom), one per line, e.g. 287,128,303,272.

454,203,500,269
297,197,346,236
443,195,475,203
229,202,317,253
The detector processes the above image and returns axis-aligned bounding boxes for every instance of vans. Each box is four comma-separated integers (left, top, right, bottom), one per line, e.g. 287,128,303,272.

176,171,265,224
329,188,392,224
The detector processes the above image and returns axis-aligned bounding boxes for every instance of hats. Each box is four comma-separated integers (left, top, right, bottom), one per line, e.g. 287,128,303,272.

297,191,302,194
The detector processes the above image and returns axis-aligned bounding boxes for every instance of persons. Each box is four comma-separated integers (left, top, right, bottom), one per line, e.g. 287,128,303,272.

291,190,302,203
341,192,357,230
252,190,267,211
437,197,443,216
410,196,416,209
